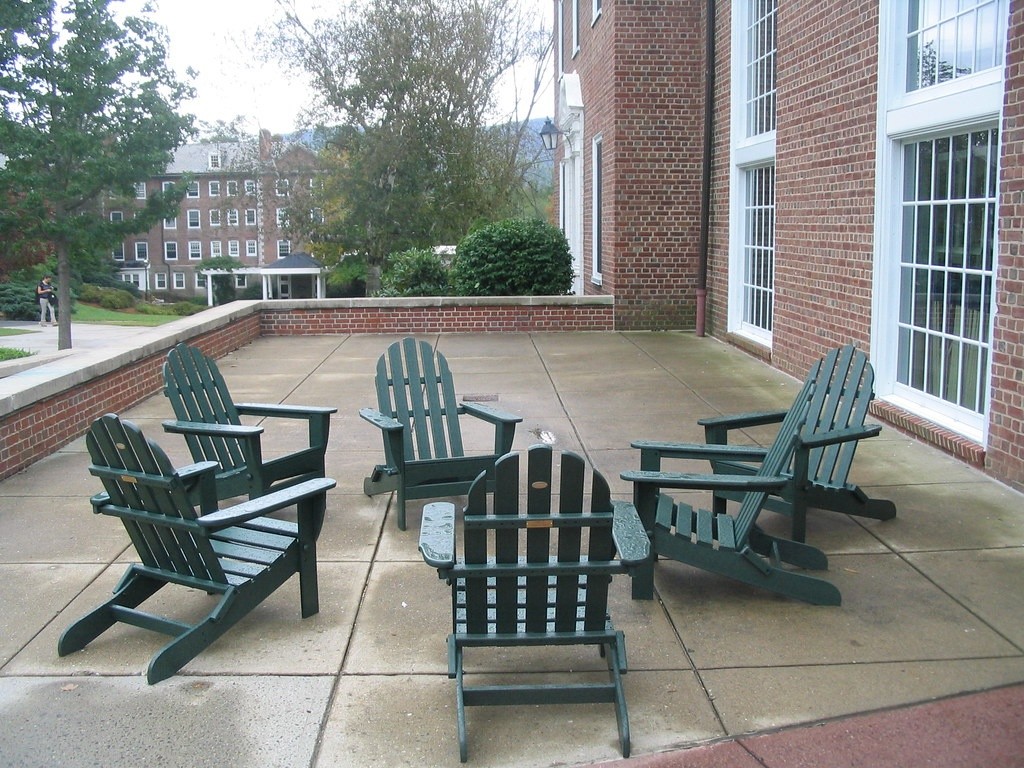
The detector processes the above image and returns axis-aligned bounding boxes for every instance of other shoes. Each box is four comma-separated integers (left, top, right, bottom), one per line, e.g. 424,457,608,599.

41,322,47,327
52,322,58,327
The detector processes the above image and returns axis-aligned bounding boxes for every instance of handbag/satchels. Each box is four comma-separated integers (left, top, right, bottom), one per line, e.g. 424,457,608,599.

49,292,57,306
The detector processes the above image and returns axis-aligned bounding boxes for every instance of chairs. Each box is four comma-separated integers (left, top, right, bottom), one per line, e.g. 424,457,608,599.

353,334,525,533
620,375,844,611
50,409,338,685
153,339,341,513
413,440,652,764
694,341,896,541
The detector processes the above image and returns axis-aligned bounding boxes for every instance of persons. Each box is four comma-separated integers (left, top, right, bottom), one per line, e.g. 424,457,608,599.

38,274,58,326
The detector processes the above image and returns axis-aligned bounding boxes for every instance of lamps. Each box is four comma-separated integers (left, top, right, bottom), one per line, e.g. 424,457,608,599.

538,115,575,161
